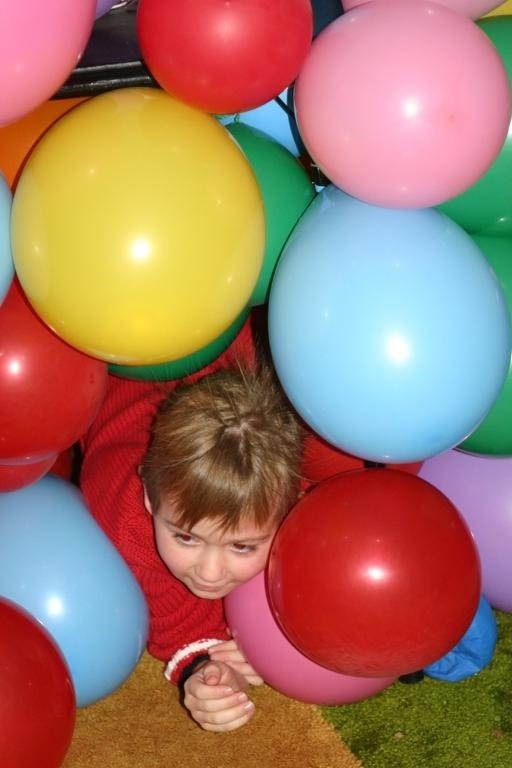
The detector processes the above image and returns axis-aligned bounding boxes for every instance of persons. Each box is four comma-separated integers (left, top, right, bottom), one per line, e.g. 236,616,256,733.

79,313,320,733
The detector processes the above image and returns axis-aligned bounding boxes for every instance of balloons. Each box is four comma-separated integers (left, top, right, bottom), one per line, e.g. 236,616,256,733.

0,453,58,495
416,446,512,614
300,428,423,484
0,595,77,768
220,122,318,308
434,137,512,235
0,96,97,197
0,0,96,127
108,304,252,384
224,568,397,706
0,473,149,709
423,592,497,683
217,80,328,194
1,272,109,466
341,0,512,22
135,0,313,115
456,234,512,458
95,0,122,18
474,15,512,93
10,86,267,366
267,183,512,465
478,0,512,20
0,170,15,310
293,0,512,208
264,467,481,678
